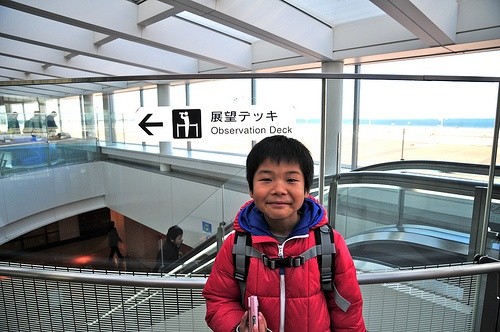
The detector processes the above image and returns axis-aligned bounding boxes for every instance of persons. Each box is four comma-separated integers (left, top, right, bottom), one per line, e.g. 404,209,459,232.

202,135,368,332
7,111,21,137
29,110,46,138
42,111,59,138
108,221,126,261
151,225,183,274
